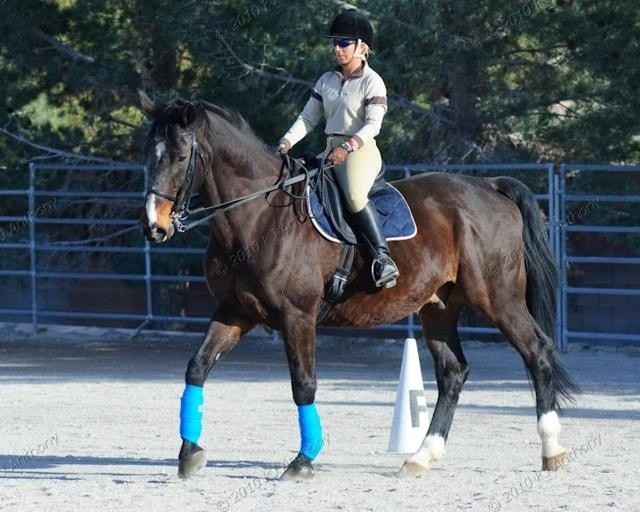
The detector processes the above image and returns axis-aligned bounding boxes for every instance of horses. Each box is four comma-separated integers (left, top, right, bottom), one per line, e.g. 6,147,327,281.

135,87,583,484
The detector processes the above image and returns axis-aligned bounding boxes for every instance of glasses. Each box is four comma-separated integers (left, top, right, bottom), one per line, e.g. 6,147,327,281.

331,39,356,47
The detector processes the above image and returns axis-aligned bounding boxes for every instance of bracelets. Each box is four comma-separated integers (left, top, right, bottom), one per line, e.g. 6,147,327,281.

340,138,357,154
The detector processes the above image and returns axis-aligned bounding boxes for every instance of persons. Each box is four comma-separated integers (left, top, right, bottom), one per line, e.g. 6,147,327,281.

275,8,397,288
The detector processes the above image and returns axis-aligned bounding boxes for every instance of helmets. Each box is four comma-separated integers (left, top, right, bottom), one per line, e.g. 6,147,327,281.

323,9,373,48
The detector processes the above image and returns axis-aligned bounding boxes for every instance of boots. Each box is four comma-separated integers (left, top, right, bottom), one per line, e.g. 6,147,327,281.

352,201,396,289
